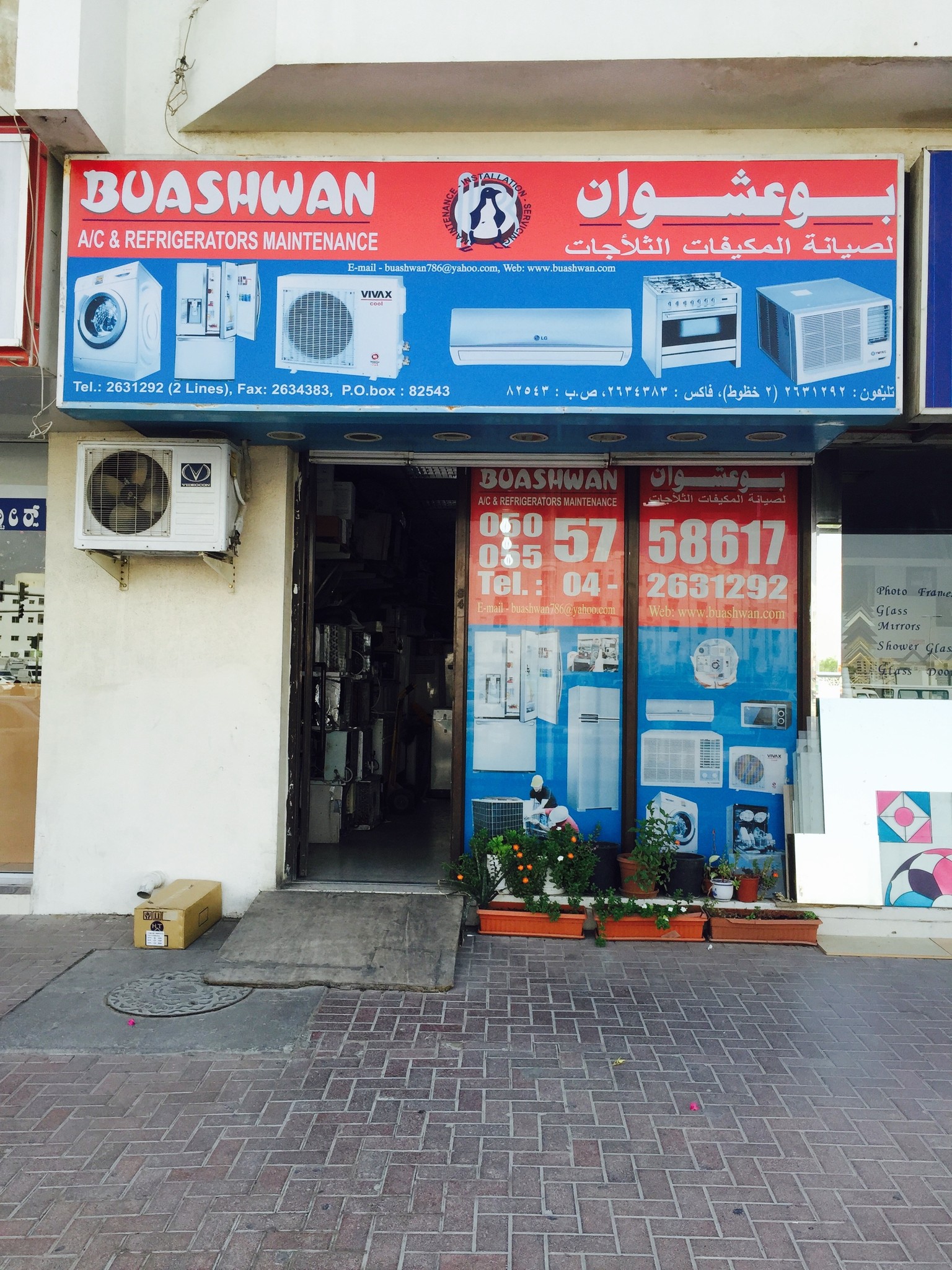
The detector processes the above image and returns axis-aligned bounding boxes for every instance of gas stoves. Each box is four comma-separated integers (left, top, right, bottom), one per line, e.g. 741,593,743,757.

642,272,741,297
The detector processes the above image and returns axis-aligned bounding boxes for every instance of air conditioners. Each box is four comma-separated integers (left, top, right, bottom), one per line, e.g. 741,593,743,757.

754,277,892,386
448,307,633,367
641,729,723,788
408,655,441,715
644,698,716,724
274,273,407,381
728,745,788,795
72,440,242,557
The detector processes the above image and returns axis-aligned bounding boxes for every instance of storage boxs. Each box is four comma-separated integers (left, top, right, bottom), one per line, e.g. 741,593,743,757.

133,878,222,951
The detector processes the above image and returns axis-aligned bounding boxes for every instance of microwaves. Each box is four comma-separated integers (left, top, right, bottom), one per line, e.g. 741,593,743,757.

740,700,792,730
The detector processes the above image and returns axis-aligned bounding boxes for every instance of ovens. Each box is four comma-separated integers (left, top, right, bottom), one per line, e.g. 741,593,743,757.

642,297,742,379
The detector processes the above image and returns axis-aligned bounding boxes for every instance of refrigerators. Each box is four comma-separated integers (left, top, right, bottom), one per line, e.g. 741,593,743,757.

173,260,261,382
470,632,563,770
566,685,619,813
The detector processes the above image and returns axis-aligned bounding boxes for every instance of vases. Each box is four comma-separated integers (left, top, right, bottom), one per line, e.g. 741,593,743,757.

734,873,759,903
666,851,706,898
537,855,565,895
592,904,708,939
486,854,516,894
476,900,588,936
617,852,656,893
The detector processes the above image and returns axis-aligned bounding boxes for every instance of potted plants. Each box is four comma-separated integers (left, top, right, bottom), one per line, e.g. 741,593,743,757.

702,897,823,946
704,841,740,901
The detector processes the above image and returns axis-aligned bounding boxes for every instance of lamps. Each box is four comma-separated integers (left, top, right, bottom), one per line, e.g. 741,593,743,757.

609,451,816,466
309,450,610,469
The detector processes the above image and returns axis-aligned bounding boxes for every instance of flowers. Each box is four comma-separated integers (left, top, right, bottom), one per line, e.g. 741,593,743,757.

438,821,602,923
588,883,694,948
624,800,680,893
738,855,782,901
